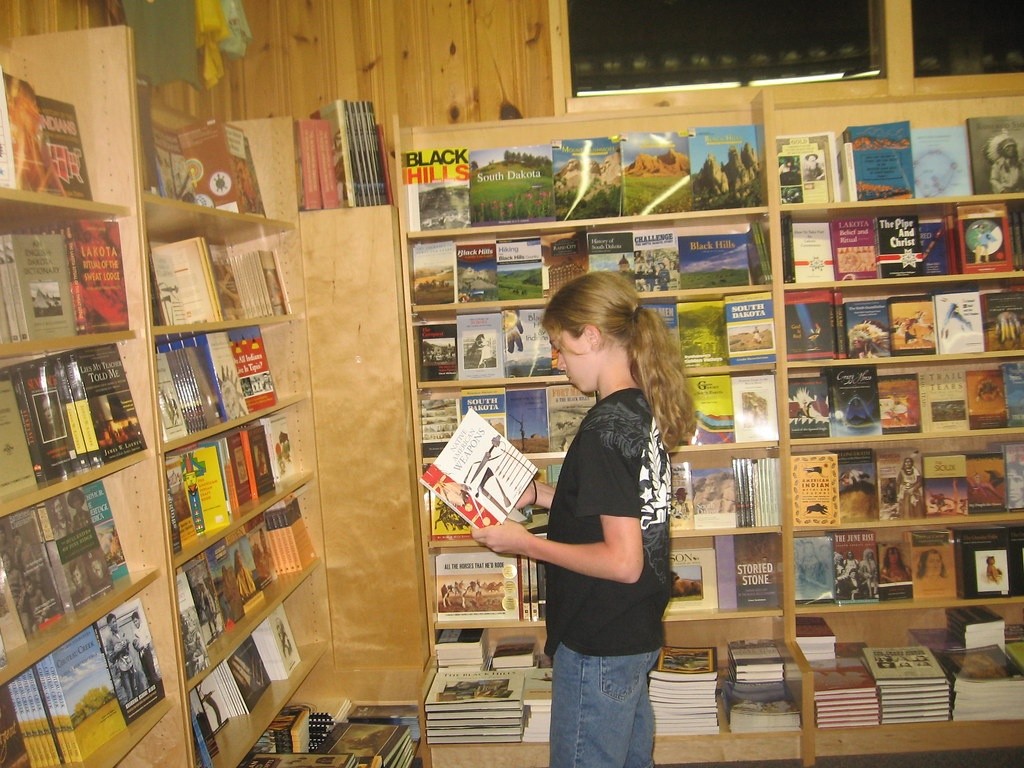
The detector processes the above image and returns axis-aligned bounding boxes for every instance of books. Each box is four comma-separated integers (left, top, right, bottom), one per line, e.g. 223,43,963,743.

394,119,1024,744
137,98,393,766
0,63,168,767
239,700,420,768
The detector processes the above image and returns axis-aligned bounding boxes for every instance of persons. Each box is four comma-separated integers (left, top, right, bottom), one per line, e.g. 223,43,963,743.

476,270,700,768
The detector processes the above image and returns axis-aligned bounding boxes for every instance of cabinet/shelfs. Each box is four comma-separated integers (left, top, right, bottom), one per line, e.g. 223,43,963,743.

0,174,1024,768
